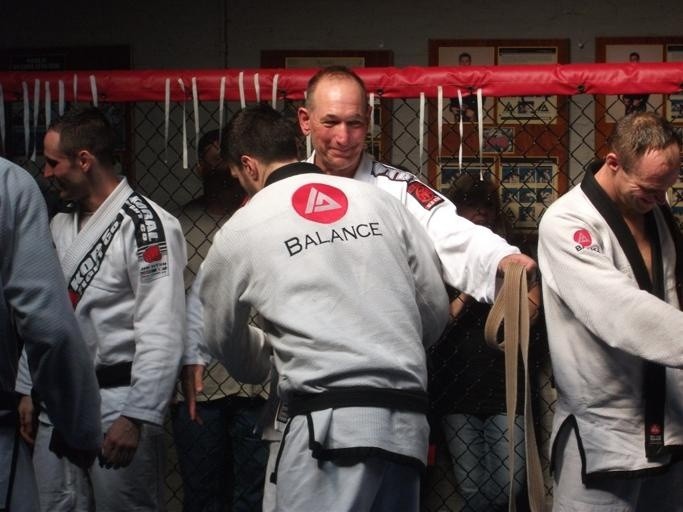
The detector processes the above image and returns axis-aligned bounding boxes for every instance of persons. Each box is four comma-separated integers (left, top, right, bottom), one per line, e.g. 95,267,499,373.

185,63,682,511
617,52,650,115
449,53,487,120
1,106,189,511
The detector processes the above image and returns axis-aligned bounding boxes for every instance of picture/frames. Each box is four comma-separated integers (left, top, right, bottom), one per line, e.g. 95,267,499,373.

427,37,570,238
0,43,133,158
257,49,393,167
595,36,681,218
